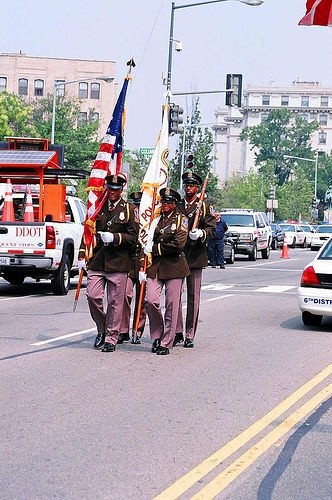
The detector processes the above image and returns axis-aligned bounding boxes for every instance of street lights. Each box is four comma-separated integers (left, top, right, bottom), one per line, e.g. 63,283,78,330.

283,152,318,199
51,75,115,145
165,0,265,103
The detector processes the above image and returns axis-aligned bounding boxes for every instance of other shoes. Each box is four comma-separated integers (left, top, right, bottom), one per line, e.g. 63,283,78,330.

118,333,130,344
174,334,184,347
212,265,216,268
184,339,194,347
132,336,141,344
220,264,225,269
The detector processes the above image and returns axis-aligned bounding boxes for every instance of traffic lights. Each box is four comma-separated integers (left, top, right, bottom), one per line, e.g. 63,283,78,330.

185,154,194,169
170,103,184,137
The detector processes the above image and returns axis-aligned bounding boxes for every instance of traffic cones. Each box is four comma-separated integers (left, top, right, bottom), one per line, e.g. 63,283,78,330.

18,188,45,226
0,178,21,225
280,237,290,259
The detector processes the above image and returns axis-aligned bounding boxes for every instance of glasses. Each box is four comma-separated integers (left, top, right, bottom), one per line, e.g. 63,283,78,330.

160,199,173,204
185,184,196,188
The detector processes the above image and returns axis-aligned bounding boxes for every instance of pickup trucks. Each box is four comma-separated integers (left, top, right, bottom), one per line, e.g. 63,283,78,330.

0,184,87,295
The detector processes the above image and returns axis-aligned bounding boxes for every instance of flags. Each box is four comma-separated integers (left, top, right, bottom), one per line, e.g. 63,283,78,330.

83,78,128,261
138,106,170,268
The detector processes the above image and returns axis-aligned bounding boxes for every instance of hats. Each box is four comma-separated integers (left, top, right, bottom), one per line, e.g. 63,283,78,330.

129,191,143,204
160,188,181,204
182,172,202,185
105,175,127,190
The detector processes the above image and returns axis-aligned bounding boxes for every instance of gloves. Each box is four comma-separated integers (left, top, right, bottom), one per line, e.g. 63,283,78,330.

96,231,114,243
145,244,152,252
78,258,86,275
189,229,203,240
139,272,147,285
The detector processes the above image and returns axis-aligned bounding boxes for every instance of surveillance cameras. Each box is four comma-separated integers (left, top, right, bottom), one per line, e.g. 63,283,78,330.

175,41,182,51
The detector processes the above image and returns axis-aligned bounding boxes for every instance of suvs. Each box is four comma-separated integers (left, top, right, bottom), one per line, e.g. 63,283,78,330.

218,208,273,261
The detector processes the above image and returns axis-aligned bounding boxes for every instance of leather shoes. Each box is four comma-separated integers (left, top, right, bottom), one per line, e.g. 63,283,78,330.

95,333,105,348
152,339,169,355
102,343,115,352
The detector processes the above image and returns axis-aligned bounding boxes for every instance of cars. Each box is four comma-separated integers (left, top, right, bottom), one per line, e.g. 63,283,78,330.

300,225,315,248
270,223,285,250
311,225,332,251
204,233,236,264
278,223,306,248
298,236,332,326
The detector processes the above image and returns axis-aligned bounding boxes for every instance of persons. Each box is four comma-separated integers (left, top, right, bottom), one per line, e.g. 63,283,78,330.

207,210,228,269
139,188,191,356
174,172,216,348
77,175,138,352
116,192,146,344
